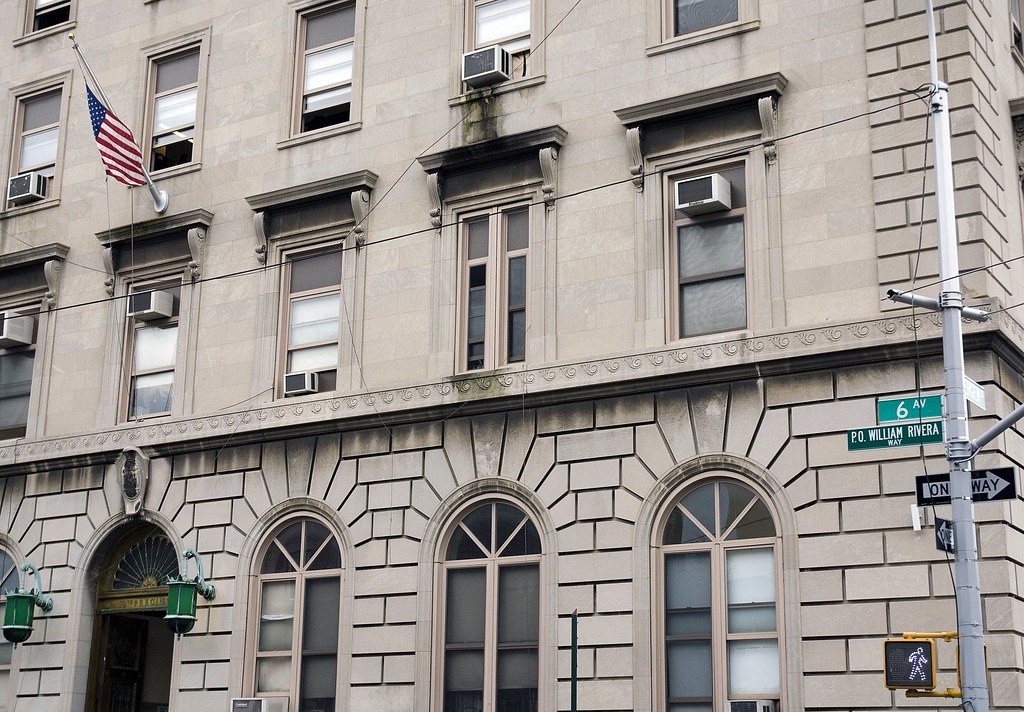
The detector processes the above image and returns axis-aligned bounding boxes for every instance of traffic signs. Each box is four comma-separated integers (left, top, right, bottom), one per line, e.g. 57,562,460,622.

932,515,983,557
913,466,1018,507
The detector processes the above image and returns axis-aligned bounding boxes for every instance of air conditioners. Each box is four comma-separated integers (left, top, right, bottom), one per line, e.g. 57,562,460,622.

7,172,48,206
723,699,775,712
284,372,318,394
0,310,35,349
461,44,512,89
126,289,174,322
674,173,732,218
230,697,288,712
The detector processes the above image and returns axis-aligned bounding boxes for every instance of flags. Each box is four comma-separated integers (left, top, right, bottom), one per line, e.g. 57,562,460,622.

86,84,147,187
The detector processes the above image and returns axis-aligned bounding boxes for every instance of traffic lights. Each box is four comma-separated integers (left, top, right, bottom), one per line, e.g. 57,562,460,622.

881,639,937,691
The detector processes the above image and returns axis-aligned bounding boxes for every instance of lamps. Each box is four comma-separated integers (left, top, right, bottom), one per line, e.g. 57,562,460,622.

1,562,53,650
163,549,216,642
